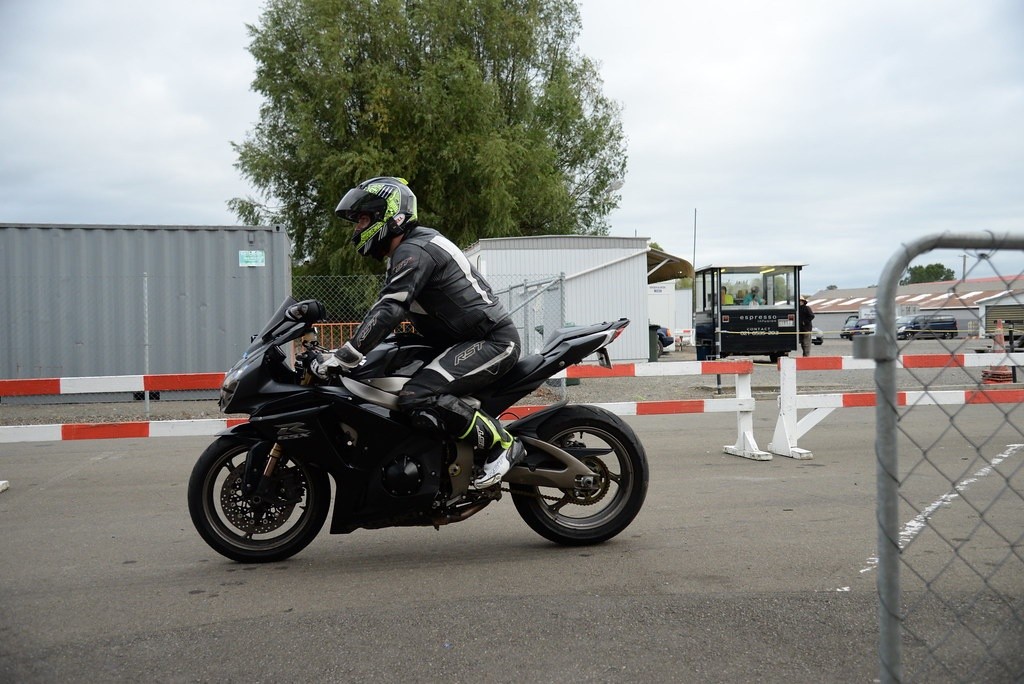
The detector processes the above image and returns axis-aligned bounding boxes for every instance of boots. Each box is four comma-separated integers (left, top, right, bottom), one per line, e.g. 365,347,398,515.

459,408,528,490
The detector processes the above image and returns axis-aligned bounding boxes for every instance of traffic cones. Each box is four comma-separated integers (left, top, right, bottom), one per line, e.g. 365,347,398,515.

982,319,1015,381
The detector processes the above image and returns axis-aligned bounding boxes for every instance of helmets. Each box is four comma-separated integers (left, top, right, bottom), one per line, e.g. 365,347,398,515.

335,176,419,262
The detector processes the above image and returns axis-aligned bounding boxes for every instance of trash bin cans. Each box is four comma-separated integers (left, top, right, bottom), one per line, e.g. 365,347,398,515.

649,324,660,362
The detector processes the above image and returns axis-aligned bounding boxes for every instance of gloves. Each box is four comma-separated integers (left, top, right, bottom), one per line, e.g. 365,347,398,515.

310,341,367,381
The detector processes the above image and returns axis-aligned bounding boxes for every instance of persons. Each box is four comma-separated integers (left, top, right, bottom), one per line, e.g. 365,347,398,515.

312,177,529,495
714,284,765,306
797,295,814,356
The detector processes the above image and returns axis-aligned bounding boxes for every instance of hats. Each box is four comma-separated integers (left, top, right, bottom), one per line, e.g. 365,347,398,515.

751,286,759,294
800,295,808,304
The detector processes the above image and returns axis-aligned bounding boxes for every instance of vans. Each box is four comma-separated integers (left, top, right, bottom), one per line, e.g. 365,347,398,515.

896,314,958,340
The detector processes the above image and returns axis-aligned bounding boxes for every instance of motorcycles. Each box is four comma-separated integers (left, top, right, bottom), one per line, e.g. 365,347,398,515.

809,328,824,345
188,295,649,565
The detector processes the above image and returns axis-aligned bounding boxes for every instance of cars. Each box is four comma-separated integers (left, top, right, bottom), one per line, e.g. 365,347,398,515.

655,327,674,357
840,316,902,340
